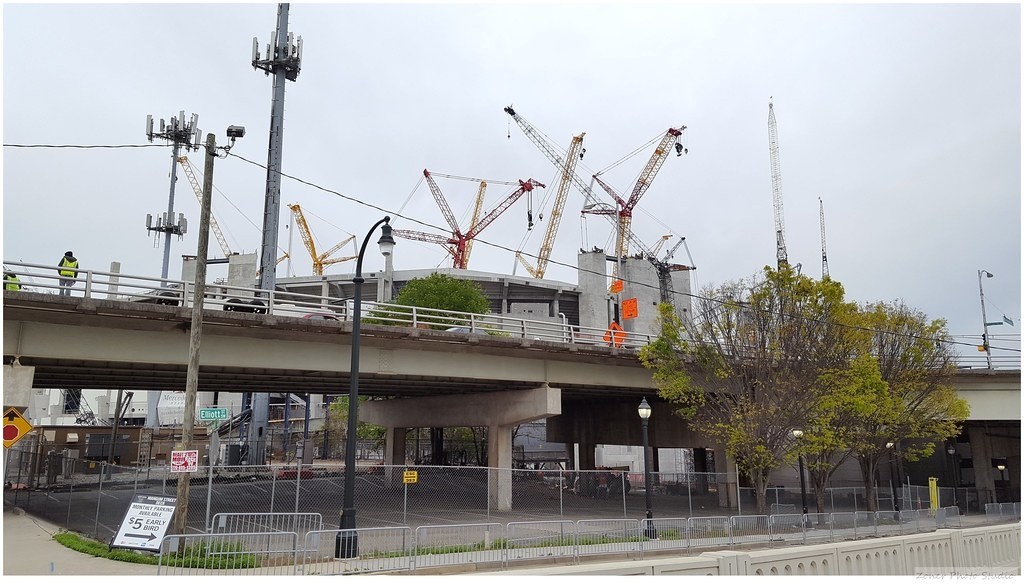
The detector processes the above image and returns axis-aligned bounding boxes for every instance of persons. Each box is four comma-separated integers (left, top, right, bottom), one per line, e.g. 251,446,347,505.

3,270,22,291
58,251,78,296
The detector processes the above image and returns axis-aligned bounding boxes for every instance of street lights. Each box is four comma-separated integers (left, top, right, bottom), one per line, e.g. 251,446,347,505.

977,269,994,371
947,444,964,515
329,215,396,563
885,439,903,520
997,460,1009,503
791,426,813,528
637,396,658,537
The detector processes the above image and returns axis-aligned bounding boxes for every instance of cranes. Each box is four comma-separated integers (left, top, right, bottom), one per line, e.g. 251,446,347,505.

766,94,791,271
816,196,833,278
179,102,699,325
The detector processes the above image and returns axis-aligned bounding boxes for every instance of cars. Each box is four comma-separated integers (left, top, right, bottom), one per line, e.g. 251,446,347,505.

303,313,340,320
447,327,491,335
